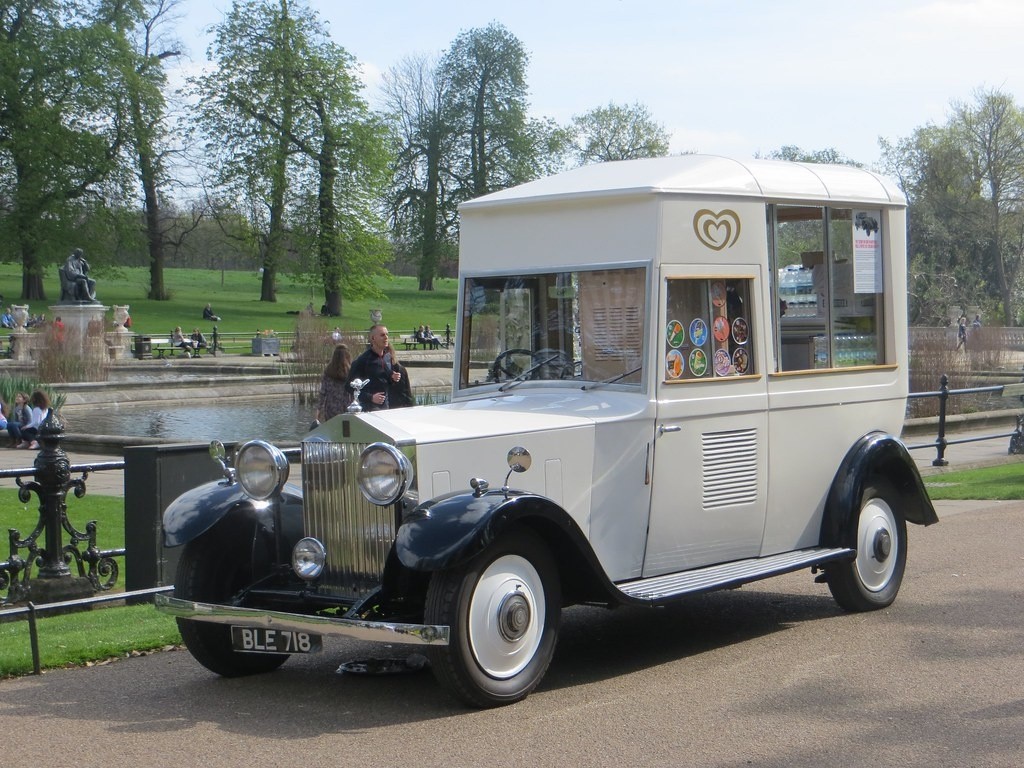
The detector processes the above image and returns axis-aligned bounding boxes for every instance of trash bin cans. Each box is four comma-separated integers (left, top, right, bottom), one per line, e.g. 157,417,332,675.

130,335,153,360
14,306,28,327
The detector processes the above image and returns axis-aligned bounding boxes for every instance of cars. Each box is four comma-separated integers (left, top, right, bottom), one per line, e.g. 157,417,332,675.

152,154,938,709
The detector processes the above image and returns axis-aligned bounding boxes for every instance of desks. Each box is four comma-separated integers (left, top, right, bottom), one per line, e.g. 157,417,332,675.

251,337,281,357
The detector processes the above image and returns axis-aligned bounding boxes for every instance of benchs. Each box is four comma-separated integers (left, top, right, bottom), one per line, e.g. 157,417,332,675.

400,334,447,350
151,338,213,358
325,335,370,349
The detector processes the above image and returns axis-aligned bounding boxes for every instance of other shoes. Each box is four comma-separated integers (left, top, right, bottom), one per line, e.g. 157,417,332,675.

184,351,191,359
6,440,20,448
17,440,29,448
28,441,40,449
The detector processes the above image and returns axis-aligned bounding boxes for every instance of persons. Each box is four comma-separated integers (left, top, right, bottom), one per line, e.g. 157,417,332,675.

1,307,47,330
0,389,54,450
203,303,221,321
173,326,207,358
956,317,968,354
51,316,65,350
972,314,981,335
111,316,131,328
315,324,413,424
417,325,446,350
65,248,96,301
